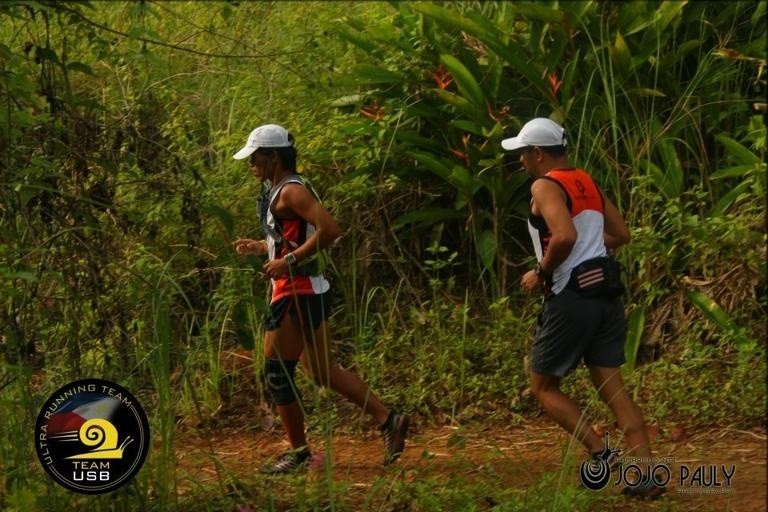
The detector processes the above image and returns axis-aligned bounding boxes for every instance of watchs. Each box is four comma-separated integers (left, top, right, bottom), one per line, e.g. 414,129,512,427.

284,252,298,268
534,263,551,281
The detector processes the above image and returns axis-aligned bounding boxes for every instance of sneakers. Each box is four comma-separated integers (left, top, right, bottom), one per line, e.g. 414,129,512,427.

621,475,668,496
262,448,312,475
579,449,623,488
379,415,410,466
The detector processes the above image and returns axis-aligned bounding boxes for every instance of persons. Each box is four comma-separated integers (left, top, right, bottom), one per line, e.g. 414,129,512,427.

501,117,667,501
232,124,409,477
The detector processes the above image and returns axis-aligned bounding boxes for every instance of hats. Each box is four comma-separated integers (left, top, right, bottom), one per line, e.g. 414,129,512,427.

501,117,567,151
232,124,293,160
